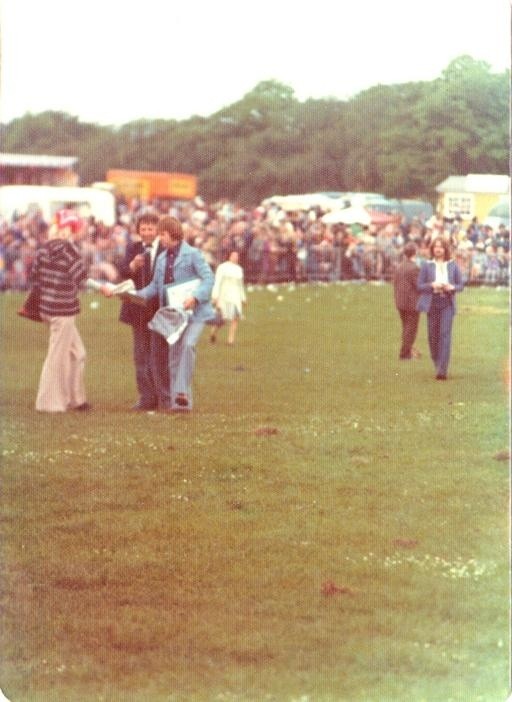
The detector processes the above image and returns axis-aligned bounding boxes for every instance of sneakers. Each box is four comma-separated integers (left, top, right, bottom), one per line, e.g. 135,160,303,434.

175,394,189,406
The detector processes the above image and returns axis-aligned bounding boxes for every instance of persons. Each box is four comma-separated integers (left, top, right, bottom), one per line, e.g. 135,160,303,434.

119,217,215,414
207,250,246,349
116,211,171,417
2,193,510,293
27,210,112,413
412,235,464,379
393,241,423,360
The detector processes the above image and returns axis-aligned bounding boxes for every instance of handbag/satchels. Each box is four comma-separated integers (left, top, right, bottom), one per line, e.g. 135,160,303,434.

206,306,223,327
17,287,44,322
